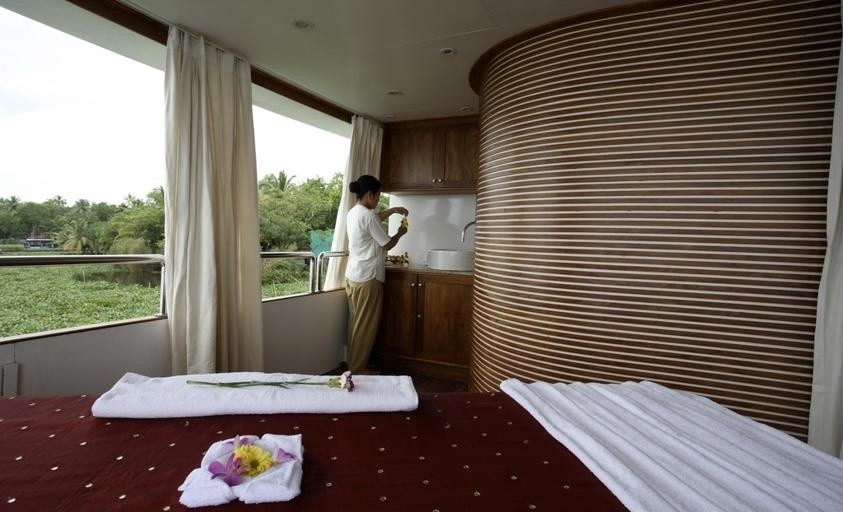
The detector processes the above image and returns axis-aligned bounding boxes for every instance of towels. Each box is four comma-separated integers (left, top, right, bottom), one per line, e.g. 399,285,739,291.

175,433,304,506
496,365,843,510
90,372,419,418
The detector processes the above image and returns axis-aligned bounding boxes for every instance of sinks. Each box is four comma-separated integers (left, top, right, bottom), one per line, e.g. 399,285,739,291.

426,249,474,272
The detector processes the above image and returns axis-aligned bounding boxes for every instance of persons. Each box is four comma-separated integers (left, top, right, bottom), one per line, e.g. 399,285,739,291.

345,176,408,375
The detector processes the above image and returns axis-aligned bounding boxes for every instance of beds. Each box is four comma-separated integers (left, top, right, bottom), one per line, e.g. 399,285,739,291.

2,393,626,510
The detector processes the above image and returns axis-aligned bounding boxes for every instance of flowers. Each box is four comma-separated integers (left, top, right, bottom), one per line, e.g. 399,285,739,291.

184,371,357,392
213,434,291,482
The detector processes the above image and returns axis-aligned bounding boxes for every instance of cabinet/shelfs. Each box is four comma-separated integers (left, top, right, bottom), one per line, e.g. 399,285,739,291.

380,116,478,196
368,272,474,379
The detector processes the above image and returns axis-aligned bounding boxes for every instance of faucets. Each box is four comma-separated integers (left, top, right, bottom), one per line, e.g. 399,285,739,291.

460,220,476,243
